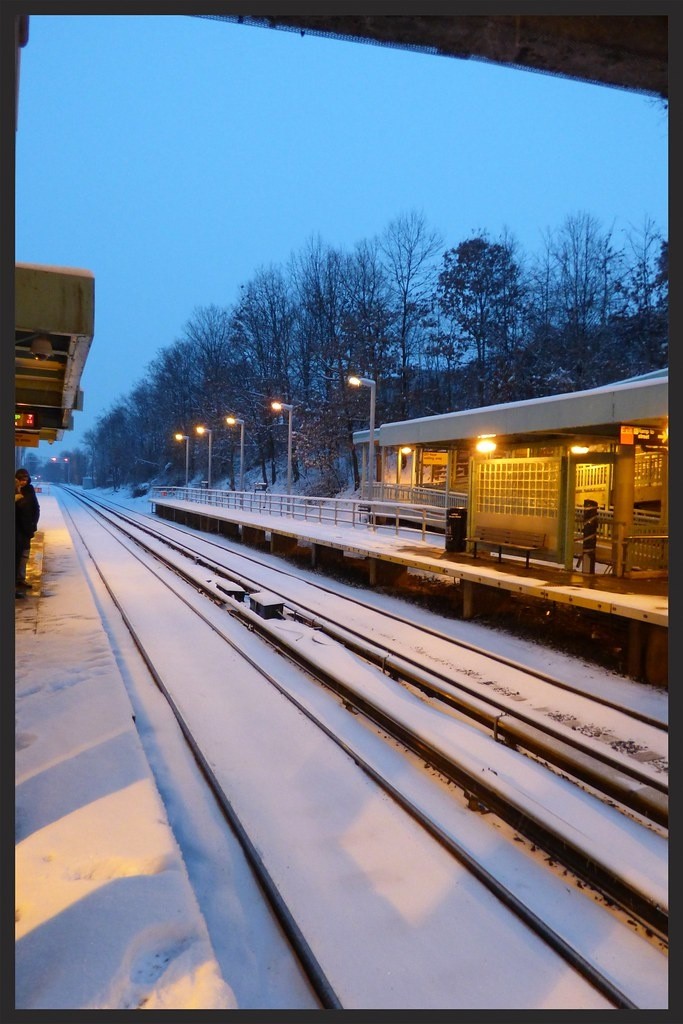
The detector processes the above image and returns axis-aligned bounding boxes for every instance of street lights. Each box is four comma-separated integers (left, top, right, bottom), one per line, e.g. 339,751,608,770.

227,417,245,510
347,376,376,502
271,401,294,513
196,426,213,504
175,435,189,499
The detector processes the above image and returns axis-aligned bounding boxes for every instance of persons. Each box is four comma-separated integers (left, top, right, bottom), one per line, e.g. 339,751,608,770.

15,469,40,598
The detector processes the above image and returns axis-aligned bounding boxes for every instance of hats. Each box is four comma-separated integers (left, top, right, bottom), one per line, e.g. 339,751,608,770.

15,469,29,480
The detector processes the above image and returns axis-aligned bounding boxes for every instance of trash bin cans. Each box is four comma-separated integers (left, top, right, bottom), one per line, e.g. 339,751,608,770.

446,506,467,553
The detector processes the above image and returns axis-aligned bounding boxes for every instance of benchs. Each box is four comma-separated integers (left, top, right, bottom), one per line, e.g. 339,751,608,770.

463,525,546,569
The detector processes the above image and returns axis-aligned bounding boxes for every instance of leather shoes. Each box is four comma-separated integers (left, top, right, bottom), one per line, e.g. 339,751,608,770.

21,581,33,589
15,590,25,599
